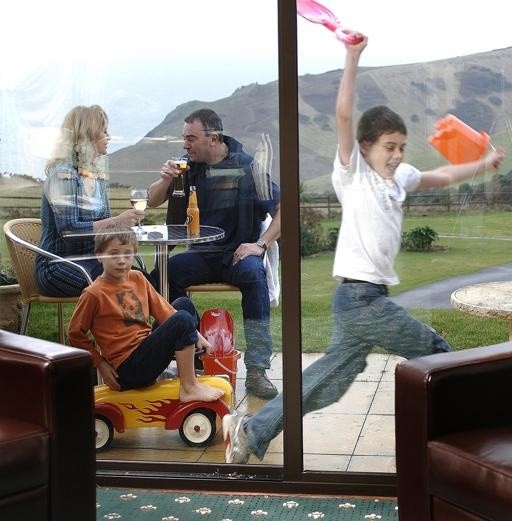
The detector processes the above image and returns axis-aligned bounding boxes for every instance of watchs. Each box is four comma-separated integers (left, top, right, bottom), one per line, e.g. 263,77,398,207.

257,237,268,250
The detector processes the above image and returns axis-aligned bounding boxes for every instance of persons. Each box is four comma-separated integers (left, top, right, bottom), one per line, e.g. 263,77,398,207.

148,108,284,400
221,31,504,464
65,218,226,403
33,103,157,298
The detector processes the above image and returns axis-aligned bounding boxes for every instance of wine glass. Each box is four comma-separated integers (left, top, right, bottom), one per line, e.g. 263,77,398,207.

129,187,148,235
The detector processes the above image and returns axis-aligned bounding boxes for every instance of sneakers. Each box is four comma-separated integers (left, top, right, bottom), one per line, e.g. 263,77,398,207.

245,367,278,398
223,414,254,464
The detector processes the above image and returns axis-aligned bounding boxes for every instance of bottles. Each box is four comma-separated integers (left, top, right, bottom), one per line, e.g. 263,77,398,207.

184,184,200,234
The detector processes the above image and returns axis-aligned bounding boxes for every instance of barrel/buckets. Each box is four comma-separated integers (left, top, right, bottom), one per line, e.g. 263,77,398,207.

198,349,242,394
428,113,496,166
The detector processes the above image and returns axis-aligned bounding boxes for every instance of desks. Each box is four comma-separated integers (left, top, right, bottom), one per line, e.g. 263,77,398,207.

124,223,227,318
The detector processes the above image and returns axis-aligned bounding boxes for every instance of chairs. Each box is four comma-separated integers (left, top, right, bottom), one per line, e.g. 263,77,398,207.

1,327,98,519
391,338,511,520
2,216,146,358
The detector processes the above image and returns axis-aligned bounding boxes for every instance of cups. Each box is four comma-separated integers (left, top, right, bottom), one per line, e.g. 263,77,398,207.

170,155,188,198
